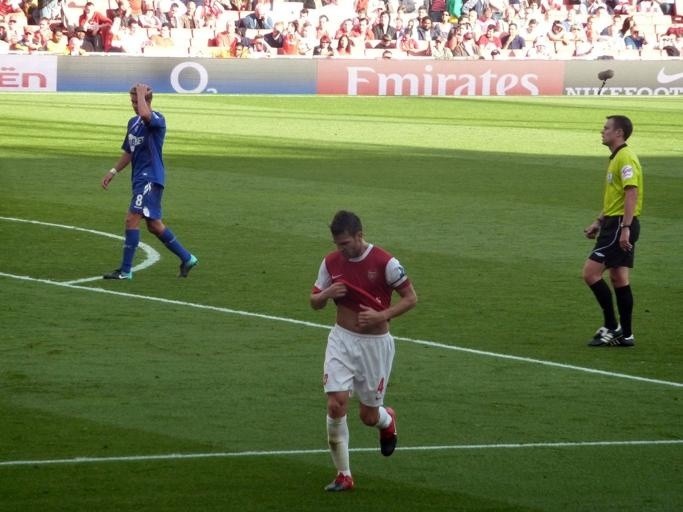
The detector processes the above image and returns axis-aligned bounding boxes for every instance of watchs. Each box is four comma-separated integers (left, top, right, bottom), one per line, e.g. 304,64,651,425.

619,222,631,228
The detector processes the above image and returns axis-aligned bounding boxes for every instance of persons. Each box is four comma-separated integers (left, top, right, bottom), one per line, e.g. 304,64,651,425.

581,115,644,345
101,84,198,280
2,0,682,60
309,210,417,492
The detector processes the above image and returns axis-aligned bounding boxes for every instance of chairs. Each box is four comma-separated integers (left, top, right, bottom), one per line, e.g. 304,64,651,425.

531,0,682,58
62,0,360,61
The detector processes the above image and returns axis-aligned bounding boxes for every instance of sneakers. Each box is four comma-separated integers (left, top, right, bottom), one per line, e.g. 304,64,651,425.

588,322,622,345
103,269,132,280
324,472,355,492
606,334,634,346
177,253,199,277
380,407,397,457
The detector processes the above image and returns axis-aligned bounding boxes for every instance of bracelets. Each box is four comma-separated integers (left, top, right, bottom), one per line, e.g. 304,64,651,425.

109,168,117,176
